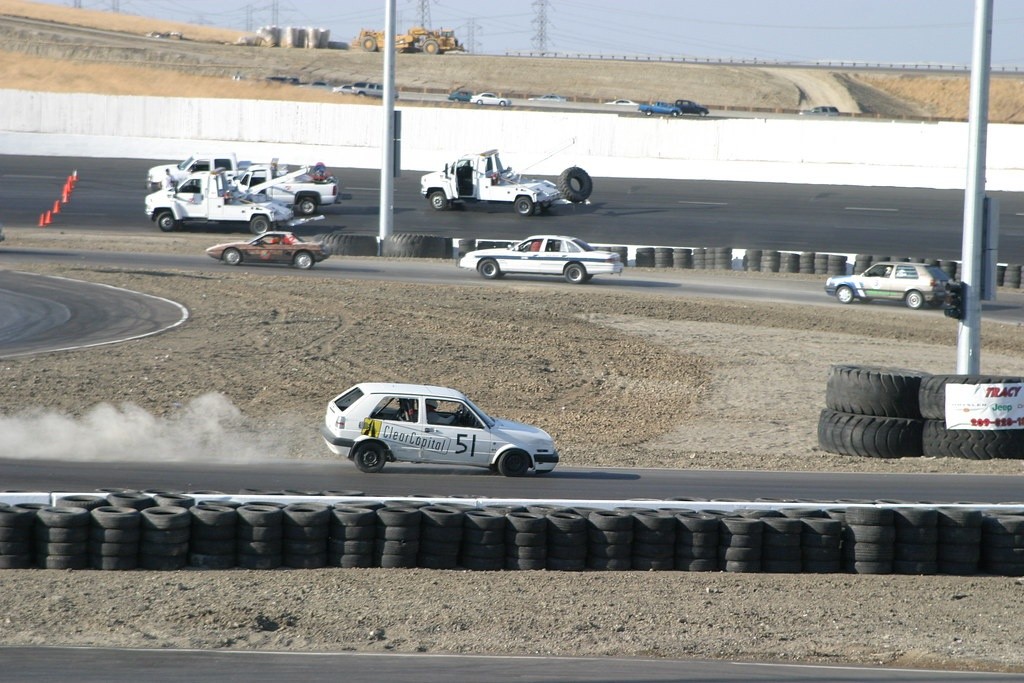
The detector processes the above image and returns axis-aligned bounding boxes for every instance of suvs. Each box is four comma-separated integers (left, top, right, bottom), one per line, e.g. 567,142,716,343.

352,81,398,99
799,106,841,116
323,383,559,477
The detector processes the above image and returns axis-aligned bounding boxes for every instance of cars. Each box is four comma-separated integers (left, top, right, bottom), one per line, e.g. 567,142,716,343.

605,99,639,106
205,230,329,270
334,83,353,95
469,93,512,107
529,94,566,101
824,262,953,310
459,235,624,284
299,81,329,88
449,91,472,102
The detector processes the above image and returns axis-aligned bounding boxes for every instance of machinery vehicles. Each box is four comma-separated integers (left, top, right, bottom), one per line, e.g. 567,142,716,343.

353,27,469,55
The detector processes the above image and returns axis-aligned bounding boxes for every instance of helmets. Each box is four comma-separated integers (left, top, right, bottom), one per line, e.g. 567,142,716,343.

426,399,438,409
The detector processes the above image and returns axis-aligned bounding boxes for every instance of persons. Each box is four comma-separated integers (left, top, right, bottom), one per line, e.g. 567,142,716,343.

426,400,461,425
884,267,892,276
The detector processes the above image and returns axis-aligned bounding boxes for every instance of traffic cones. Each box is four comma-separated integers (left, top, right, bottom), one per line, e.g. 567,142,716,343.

38,214,46,228
44,210,53,224
62,168,79,203
52,200,61,214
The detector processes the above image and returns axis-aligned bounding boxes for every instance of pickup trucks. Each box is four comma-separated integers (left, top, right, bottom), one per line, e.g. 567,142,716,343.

639,102,681,117
144,167,292,236
230,164,341,215
421,152,560,218
147,153,245,193
674,100,708,117
